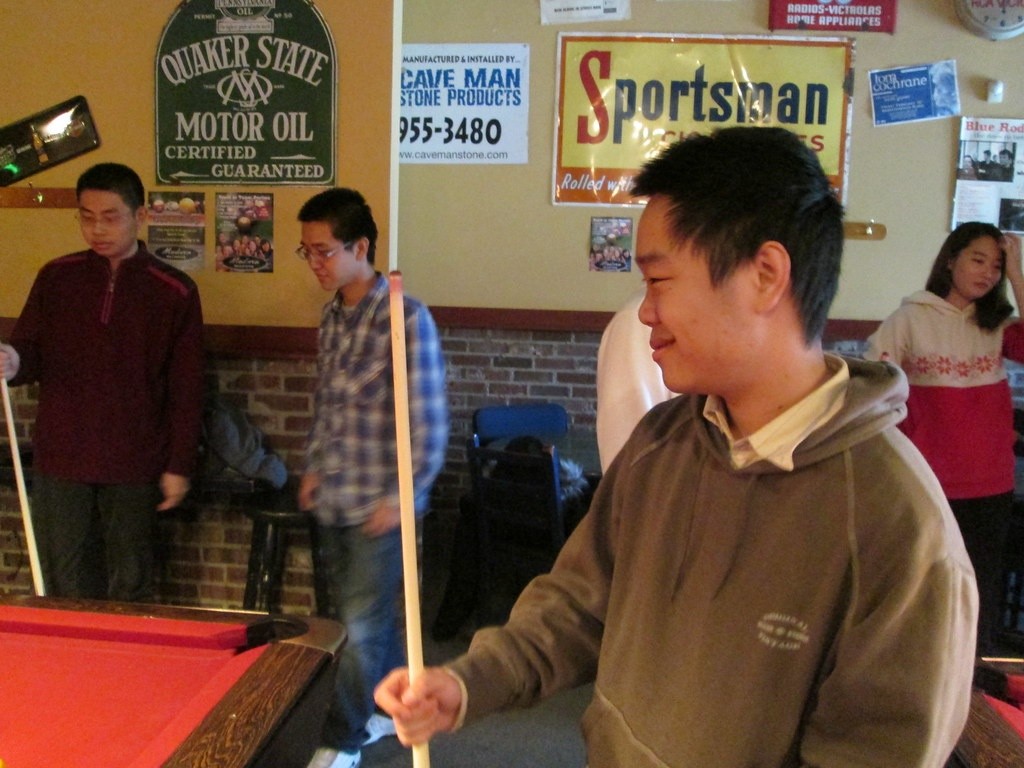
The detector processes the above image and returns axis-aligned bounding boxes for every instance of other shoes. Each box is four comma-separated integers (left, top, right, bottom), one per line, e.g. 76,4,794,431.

361,713,398,746
306,748,361,768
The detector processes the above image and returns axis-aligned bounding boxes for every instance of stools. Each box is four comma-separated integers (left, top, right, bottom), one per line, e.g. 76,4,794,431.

238,489,330,620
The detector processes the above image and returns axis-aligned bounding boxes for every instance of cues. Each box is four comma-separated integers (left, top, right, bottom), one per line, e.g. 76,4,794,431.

0,374,48,599
386,266,437,767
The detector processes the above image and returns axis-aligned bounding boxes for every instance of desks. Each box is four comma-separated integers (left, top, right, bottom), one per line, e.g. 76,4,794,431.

951,658,1024,768
0,592,348,768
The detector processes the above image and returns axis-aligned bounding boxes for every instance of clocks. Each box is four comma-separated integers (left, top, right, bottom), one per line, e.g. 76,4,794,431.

954,0,1024,41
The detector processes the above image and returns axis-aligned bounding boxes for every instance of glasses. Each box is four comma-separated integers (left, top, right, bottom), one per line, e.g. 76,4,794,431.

74,211,129,226
295,239,357,263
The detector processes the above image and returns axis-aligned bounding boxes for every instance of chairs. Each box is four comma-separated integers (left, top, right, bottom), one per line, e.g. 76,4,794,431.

433,404,602,644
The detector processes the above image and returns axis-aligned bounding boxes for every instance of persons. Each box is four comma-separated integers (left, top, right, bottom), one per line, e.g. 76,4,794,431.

0,162,206,603
296,188,450,768
371,125,981,768
217,234,273,272
598,217,1024,652
958,144,1012,181
